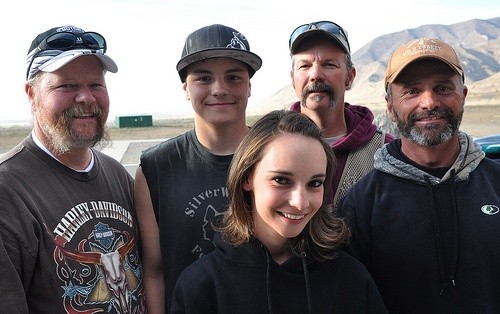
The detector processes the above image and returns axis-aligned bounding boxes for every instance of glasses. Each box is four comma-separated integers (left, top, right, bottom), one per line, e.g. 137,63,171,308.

289,21,350,52
25,31,106,55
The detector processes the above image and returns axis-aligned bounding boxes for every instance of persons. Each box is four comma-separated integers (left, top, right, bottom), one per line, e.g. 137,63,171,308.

289,20,397,217
337,36,499,313
133,23,263,314
0,25,146,314
168,109,385,314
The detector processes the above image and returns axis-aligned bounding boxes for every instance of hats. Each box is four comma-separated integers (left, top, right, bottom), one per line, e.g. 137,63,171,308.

385,38,464,92
24,25,118,80
176,24,262,83
289,18,352,56
